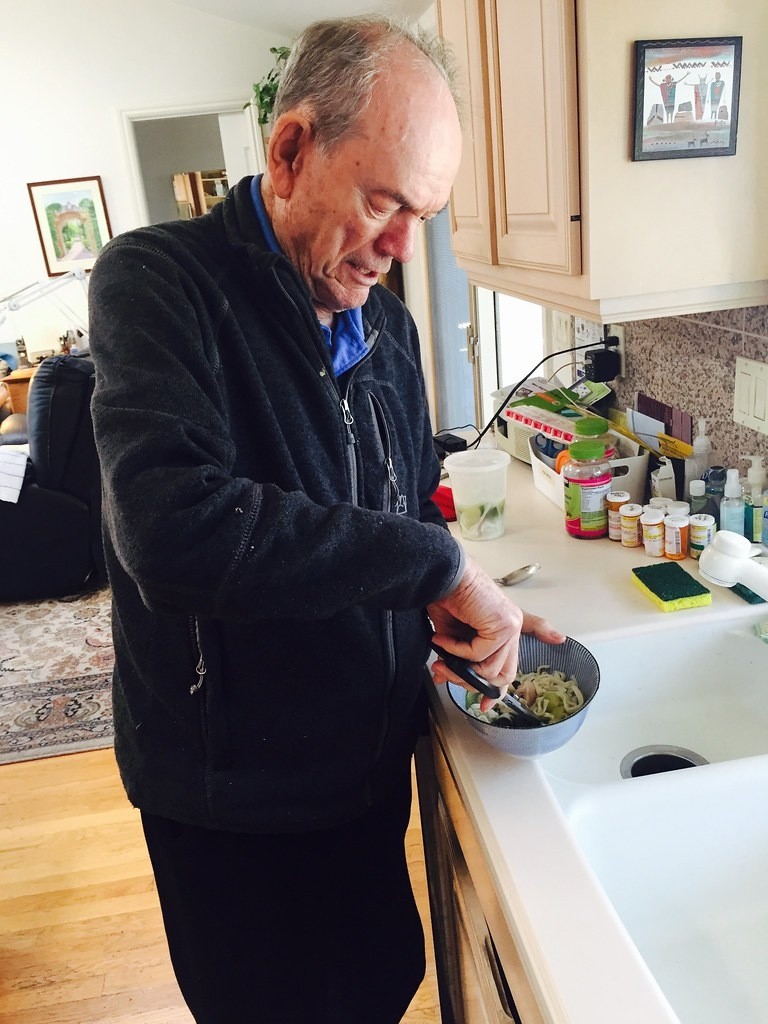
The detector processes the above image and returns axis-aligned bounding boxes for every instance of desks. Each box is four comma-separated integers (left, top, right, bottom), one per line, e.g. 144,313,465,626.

0,360,42,415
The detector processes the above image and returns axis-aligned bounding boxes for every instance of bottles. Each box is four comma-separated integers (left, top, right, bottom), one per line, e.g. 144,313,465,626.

686,479,713,515
570,418,616,477
561,441,613,540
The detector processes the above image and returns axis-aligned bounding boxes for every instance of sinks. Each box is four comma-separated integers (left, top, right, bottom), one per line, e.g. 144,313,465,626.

526,594,768,801
559,753,768,1024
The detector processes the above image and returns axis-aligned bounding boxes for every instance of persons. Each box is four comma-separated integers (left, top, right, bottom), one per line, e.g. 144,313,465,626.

86,15,567,1024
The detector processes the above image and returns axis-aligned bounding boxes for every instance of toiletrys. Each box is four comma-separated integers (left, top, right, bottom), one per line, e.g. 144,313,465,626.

683,416,726,502
739,455,768,544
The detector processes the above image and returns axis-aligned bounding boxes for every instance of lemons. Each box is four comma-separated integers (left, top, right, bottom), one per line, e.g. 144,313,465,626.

458,499,504,528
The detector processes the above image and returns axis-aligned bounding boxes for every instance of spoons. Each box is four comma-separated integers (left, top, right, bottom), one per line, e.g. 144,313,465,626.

491,563,541,587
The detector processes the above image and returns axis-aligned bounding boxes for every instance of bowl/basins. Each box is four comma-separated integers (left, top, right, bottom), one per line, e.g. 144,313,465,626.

445,633,600,757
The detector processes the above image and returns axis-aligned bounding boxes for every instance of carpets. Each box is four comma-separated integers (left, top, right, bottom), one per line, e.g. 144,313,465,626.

0,581,117,766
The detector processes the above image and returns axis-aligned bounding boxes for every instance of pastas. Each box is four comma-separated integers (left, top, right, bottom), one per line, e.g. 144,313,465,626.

518,665,584,726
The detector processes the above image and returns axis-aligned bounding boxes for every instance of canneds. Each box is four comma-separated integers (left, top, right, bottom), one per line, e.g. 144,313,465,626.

605,490,715,560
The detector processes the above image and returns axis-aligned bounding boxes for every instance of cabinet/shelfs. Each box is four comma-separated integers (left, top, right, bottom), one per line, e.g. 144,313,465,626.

411,694,547,1024
434,0,768,326
171,168,229,221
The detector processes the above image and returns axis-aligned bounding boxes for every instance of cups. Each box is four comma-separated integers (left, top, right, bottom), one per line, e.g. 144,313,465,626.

443,449,511,540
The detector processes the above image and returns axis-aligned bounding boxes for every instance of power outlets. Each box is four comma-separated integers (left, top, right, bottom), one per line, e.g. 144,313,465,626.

608,324,626,379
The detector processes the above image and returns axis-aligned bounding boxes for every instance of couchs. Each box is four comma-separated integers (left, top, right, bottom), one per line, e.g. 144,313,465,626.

0,352,109,609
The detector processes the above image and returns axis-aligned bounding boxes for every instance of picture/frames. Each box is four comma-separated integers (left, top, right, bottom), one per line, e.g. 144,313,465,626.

632,34,745,162
25,175,114,278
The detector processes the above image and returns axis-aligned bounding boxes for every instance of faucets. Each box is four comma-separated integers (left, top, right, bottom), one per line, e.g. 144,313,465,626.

698,530,767,601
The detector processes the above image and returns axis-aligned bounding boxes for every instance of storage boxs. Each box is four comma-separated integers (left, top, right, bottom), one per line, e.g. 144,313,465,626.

527,427,650,513
